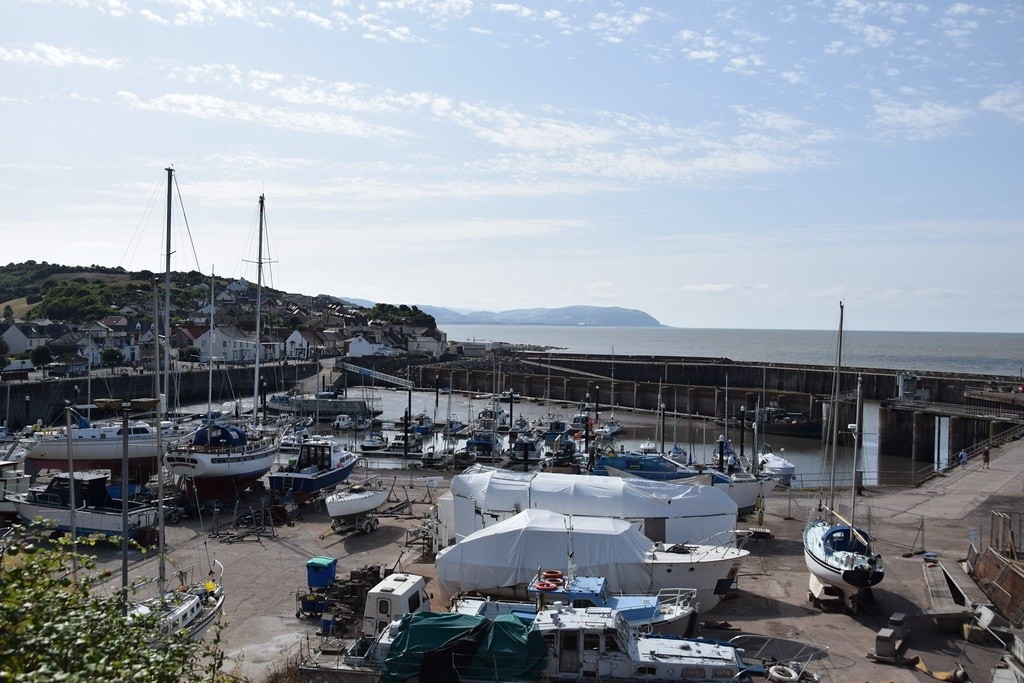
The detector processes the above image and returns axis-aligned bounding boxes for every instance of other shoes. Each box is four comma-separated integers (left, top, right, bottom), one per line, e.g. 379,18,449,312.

983,467,984,469
587,468,588,471
962,467,965,470
987,467,989,469
589,469,593,472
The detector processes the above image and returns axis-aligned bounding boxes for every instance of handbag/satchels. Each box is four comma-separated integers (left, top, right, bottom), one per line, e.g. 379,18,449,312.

982,456,985,459
959,454,962,460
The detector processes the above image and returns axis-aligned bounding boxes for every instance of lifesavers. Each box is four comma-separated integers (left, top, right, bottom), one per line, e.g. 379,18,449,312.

545,577,564,585
543,570,563,577
33,424,40,431
536,582,557,590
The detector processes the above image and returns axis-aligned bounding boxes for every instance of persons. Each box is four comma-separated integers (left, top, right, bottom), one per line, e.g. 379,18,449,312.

982,447,989,469
959,449,968,470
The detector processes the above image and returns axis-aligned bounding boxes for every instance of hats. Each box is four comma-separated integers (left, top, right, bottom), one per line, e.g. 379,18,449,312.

985,447,988,450
962,449,965,452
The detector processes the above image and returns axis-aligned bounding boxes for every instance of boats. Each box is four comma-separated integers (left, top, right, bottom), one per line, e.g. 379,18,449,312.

125,286,227,642
325,484,390,518
298,510,829,683
0,471,158,546
0,461,31,524
713,391,821,439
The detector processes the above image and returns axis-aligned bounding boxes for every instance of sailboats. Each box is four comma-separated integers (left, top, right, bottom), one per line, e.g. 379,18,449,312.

0,168,796,487
801,302,885,614
